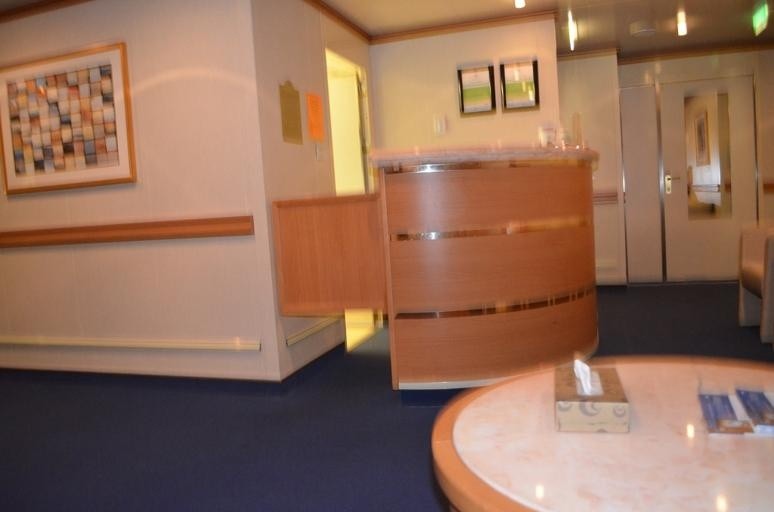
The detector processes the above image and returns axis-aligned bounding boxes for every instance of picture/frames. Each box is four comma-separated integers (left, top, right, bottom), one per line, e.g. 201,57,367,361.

457,65,496,115
500,60,540,113
0,42,139,195
694,111,710,166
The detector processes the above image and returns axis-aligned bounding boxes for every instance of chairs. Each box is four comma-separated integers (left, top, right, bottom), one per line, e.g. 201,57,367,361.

738,225,774,345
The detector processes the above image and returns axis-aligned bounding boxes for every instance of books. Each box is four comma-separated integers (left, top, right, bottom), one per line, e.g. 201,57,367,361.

735,388,774,433
698,392,753,438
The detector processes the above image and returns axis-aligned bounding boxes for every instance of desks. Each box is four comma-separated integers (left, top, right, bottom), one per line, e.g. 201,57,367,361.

431,354,774,512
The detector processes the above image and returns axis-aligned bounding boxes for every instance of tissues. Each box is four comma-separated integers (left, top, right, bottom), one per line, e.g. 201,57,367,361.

554,359,631,434
